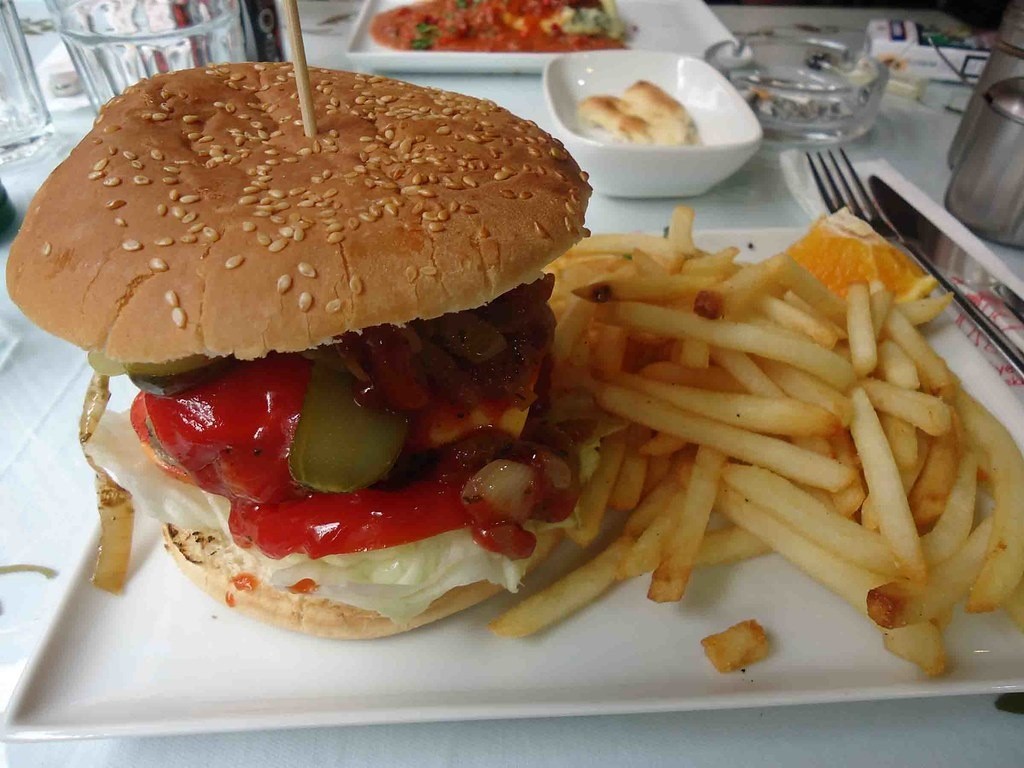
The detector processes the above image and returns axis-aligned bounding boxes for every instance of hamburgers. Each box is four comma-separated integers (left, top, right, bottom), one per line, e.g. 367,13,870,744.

2,59,590,633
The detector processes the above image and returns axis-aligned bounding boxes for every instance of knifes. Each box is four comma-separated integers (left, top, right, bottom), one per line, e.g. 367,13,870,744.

868,174,1024,325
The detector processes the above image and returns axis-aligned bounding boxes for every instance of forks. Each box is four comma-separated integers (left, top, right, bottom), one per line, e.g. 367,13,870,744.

805,148,1024,383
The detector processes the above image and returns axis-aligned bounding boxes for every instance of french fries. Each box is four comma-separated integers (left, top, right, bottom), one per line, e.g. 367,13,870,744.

549,209,1020,680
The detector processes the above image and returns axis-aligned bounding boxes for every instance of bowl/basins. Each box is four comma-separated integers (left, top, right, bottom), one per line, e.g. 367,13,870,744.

544,50,763,199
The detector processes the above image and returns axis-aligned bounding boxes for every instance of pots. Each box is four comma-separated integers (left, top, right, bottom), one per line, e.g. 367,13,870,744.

947,0,1024,170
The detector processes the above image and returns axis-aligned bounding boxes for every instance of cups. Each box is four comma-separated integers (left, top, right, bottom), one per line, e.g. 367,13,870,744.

57,0,245,115
945,76,1024,251
0,0,59,167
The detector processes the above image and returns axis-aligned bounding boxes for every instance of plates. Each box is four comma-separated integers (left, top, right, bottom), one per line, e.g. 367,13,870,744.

1,226,1024,743
344,0,738,72
